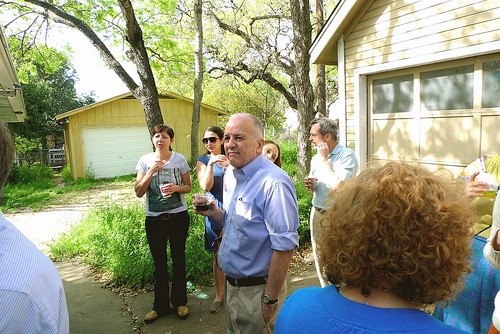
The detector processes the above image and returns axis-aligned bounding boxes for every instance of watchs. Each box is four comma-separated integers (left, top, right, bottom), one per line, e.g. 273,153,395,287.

262,291,278,305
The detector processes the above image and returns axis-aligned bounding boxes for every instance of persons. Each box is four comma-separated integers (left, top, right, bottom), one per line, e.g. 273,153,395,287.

302,118,358,287
0,119,72,334
444,152,500,334
274,162,468,334
135,124,192,323
192,112,299,333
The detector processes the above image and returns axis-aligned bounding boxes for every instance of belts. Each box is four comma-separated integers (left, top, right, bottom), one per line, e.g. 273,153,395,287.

147,212,182,222
313,205,325,214
225,275,268,287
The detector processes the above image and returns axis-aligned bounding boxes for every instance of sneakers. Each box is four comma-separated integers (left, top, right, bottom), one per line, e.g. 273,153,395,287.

209,298,224,314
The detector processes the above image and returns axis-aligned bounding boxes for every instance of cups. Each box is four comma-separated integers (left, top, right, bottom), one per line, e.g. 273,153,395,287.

307,178,316,190
194,194,211,211
159,183,172,199
216,155,226,168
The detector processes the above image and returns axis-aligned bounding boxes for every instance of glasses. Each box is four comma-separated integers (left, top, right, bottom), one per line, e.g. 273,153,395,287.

201,137,220,145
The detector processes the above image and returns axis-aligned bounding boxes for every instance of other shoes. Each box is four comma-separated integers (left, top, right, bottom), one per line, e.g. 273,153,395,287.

176,305,189,319
144,310,158,324
